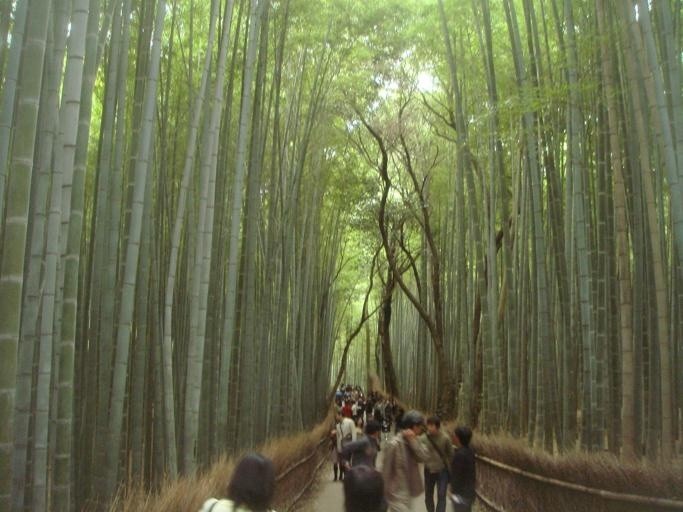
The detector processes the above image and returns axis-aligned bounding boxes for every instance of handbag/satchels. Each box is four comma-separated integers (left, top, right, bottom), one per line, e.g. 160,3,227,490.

340,433,352,447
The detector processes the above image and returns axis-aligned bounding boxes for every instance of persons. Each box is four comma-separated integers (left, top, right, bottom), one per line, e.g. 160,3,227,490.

331,383,477,512
198,452,279,512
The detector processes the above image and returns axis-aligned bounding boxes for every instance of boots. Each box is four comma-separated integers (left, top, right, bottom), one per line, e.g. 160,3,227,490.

332,467,344,482
